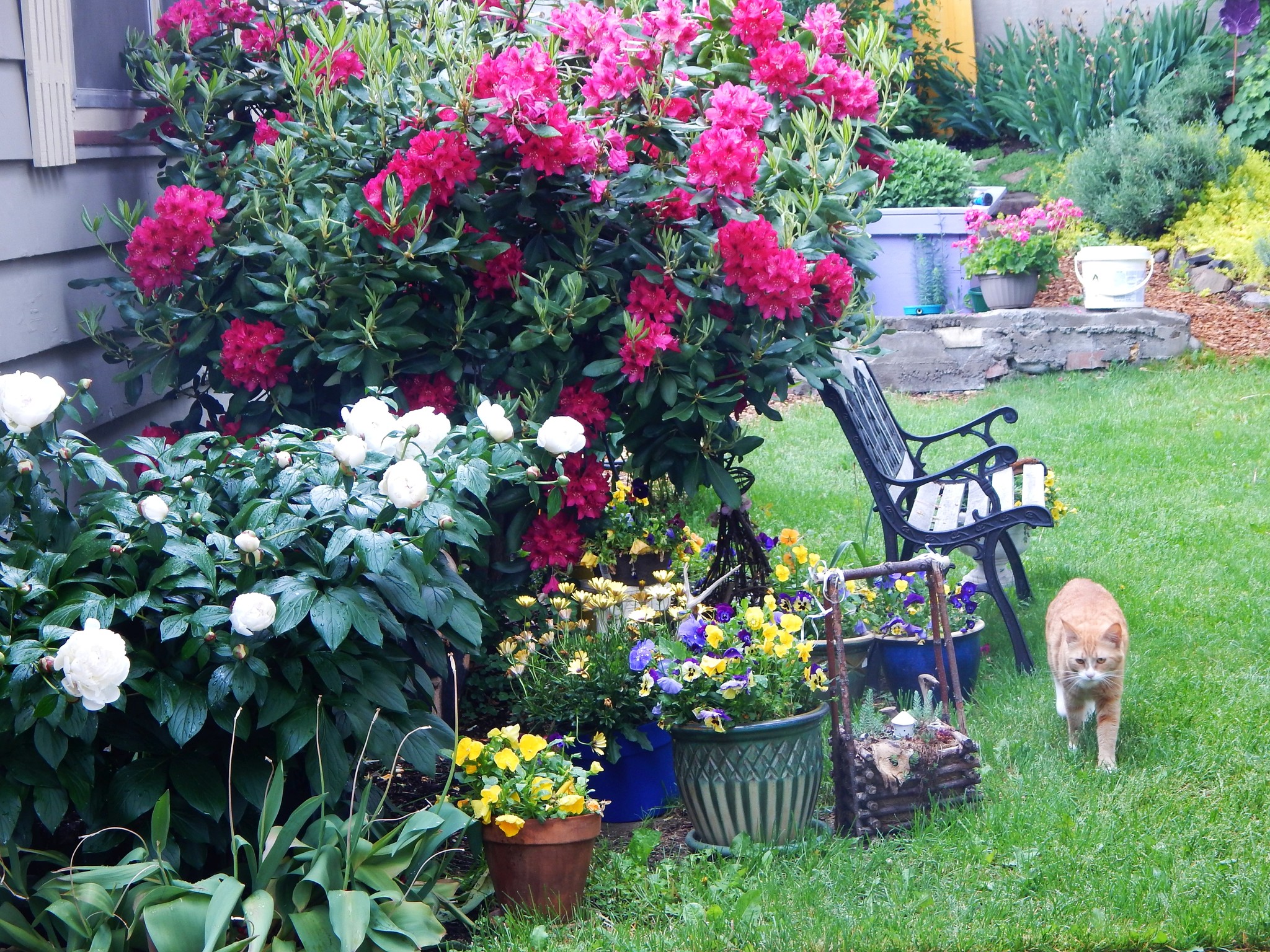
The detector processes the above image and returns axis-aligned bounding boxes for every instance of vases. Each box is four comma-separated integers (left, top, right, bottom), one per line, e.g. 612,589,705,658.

610,551,672,586
559,726,681,824
670,699,832,846
874,617,985,709
794,632,875,711
968,286,990,312
975,270,1039,309
482,816,602,920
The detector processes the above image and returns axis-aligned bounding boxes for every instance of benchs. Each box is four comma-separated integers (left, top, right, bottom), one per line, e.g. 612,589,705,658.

818,358,1056,672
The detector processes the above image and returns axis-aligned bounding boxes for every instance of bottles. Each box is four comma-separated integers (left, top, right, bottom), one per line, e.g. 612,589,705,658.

971,192,993,206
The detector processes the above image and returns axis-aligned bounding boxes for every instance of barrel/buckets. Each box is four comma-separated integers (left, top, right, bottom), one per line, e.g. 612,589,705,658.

969,286,988,313
1075,245,1154,308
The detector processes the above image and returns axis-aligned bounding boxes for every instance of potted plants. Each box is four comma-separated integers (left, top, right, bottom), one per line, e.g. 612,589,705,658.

852,137,982,315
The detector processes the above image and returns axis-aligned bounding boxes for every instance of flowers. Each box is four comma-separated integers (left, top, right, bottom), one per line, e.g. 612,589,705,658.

433,476,978,839
950,197,1083,292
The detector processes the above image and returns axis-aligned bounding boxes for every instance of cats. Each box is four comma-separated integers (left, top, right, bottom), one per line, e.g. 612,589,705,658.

1046,576,1128,774
